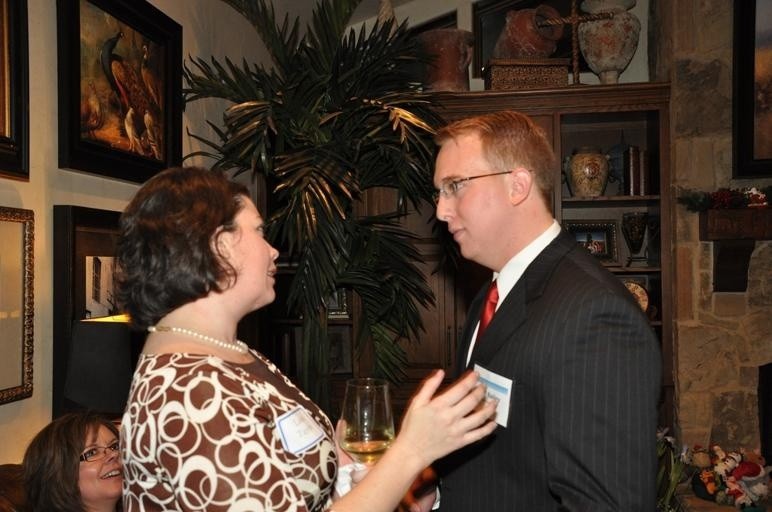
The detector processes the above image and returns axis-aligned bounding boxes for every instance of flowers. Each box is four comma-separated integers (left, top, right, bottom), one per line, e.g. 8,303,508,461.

680,183,772,211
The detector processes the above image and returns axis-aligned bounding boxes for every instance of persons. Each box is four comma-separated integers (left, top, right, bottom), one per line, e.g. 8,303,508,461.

398,112,660,512
582,234,601,255
23,413,126,511
117,167,501,512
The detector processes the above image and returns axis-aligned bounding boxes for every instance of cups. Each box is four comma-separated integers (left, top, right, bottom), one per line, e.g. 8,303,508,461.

335,377,395,466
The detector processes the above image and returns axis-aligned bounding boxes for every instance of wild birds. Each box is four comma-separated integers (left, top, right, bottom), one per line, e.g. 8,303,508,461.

101,24,161,121
81,80,103,141
139,42,162,111
143,109,163,161
124,107,142,152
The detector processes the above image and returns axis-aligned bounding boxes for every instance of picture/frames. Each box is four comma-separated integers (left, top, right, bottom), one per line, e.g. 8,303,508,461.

57,1,184,184
560,218,618,265
56,206,147,423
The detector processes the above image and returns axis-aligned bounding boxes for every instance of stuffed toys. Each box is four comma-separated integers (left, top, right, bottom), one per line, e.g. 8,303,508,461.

691,451,769,511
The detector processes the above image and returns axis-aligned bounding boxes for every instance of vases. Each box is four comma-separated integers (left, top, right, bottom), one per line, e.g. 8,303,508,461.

566,142,609,198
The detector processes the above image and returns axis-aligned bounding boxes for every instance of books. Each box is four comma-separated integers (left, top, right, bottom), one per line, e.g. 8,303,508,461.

638,151,650,192
624,145,639,196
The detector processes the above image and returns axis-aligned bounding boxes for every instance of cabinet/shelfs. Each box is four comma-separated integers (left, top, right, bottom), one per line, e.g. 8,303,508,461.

332,80,679,431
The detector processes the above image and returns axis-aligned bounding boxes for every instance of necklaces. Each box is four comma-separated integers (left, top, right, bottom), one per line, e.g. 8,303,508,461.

145,315,249,360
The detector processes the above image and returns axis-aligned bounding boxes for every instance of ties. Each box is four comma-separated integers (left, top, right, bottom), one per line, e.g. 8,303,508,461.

478,279,499,345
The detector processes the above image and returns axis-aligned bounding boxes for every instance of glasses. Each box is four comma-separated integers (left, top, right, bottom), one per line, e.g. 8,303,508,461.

77,443,119,464
430,168,511,205
